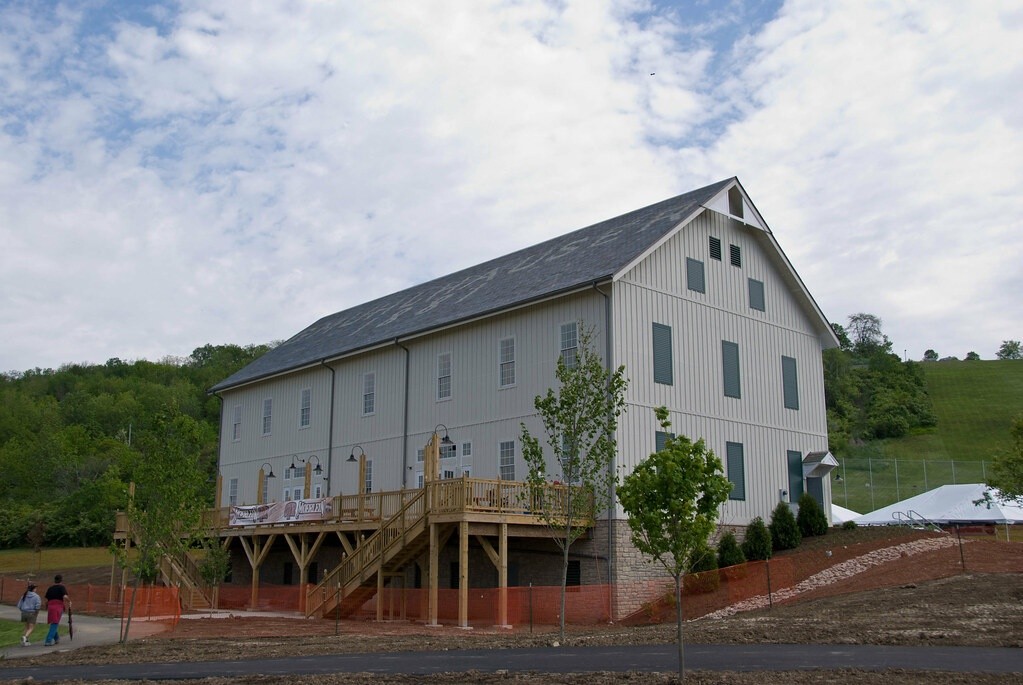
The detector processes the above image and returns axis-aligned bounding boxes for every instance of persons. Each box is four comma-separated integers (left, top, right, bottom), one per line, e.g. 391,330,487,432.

16,583,42,646
44,575,72,646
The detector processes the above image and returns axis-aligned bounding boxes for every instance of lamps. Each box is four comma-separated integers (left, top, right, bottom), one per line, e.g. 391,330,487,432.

289,454,305,469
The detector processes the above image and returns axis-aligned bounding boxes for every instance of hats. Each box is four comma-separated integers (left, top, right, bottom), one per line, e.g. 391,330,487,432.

28,583,38,590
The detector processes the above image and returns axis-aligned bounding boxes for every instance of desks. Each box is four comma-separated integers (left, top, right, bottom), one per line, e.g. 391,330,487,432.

341,508,375,517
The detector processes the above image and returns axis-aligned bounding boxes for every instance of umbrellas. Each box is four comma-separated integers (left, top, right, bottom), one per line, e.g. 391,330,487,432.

68,604,72,641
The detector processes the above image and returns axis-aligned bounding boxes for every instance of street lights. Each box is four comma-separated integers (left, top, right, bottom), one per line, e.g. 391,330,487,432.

904,350,907,362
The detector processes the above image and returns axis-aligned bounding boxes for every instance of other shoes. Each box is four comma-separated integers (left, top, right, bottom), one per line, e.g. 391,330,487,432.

20,635,30,646
55,640,60,644
45,642,54,646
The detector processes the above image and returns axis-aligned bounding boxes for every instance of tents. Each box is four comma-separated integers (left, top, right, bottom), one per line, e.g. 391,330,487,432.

832,482,1023,542
